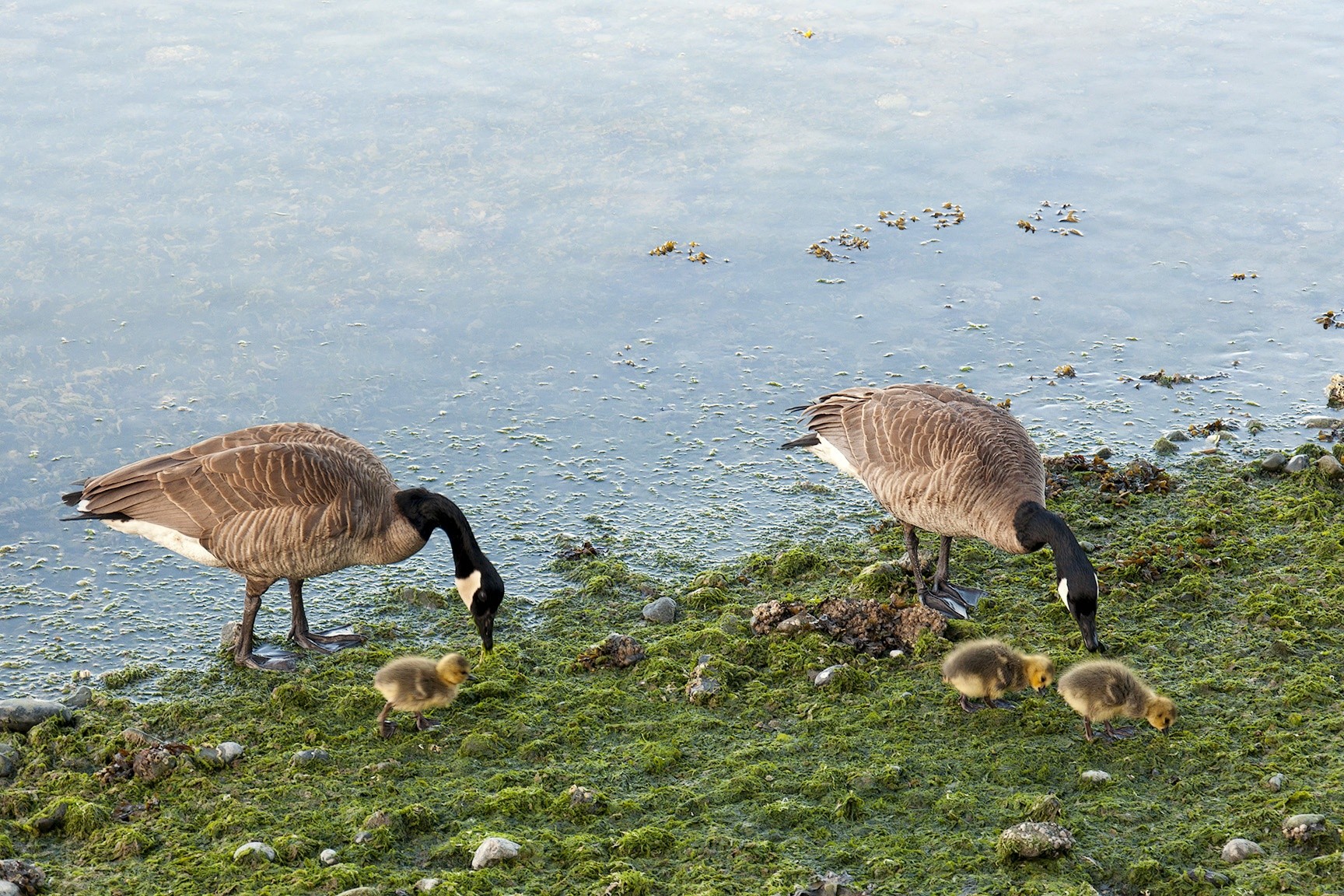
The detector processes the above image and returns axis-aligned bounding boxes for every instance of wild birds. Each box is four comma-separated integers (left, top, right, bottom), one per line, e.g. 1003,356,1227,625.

940,639,1055,714
371,652,485,739
1058,658,1179,747
58,423,506,673
777,382,1100,654
